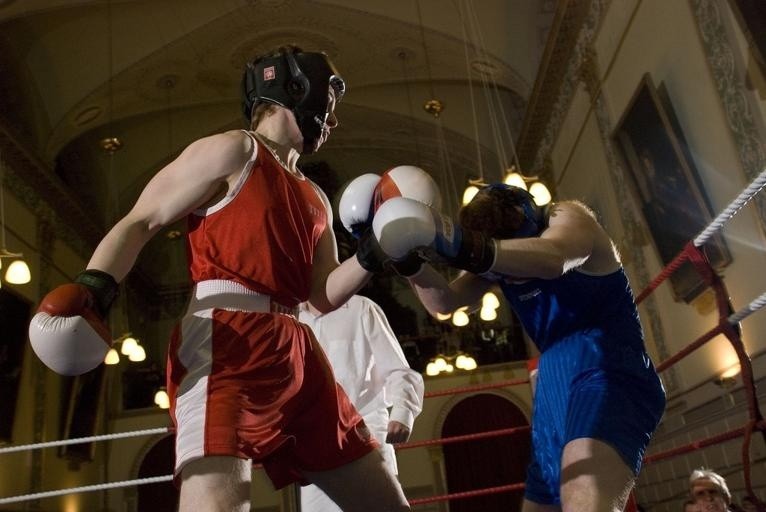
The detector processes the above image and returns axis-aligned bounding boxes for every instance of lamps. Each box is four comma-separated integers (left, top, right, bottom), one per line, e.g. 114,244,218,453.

89,0,151,369
0,221,32,290
422,100,500,378
456,1,552,210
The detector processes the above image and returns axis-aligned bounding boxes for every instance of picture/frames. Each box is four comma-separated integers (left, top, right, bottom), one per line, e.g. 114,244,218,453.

609,69,732,303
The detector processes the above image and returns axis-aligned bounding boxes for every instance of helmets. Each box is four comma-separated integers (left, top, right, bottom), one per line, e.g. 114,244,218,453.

460,183,538,237
242,46,345,154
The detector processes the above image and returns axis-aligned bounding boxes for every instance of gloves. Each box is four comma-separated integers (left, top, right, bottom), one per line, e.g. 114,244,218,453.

28,269,120,378
333,164,498,280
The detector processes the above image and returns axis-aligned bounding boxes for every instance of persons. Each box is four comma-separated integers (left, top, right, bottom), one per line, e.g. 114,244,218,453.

25,43,443,512
337,171,667,511
681,465,766,512
290,236,426,510
